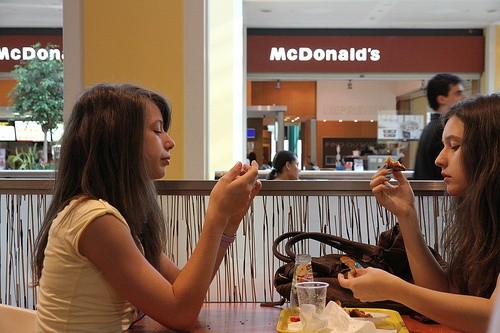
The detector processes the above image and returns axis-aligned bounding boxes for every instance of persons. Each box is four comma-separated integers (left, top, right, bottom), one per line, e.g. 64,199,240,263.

337,94,500,333
28,82,262,333
413,74,466,180
303,155,316,170
266,151,301,181
247,152,259,167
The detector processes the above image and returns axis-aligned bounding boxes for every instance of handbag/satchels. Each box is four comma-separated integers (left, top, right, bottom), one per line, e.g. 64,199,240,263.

260,223,450,314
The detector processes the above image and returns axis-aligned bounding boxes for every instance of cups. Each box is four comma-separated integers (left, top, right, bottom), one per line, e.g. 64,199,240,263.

354,159,363,171
295,281,329,328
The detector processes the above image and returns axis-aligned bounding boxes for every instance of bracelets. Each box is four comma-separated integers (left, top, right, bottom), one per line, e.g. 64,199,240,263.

221,232,236,248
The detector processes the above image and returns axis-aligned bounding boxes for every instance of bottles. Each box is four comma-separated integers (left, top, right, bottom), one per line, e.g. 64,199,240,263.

290,254,316,316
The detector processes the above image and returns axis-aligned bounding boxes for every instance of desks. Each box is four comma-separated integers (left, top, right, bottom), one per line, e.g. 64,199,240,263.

122,303,463,333
